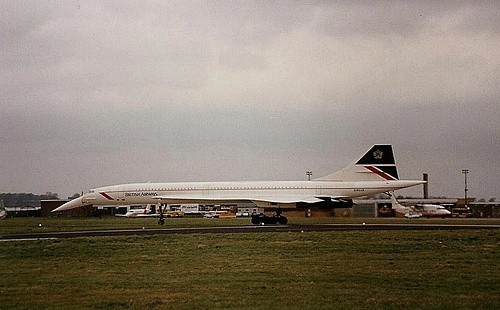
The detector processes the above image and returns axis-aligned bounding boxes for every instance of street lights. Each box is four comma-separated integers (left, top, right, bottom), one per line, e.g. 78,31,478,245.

462,169,470,205
306,172,312,180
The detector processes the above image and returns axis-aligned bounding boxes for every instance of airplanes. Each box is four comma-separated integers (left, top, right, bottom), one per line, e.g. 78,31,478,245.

50,144,427,227
387,192,451,219
